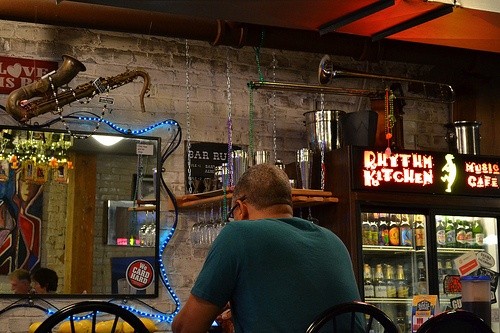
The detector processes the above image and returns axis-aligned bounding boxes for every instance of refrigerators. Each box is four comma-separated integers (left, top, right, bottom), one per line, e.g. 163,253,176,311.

285,149,500,333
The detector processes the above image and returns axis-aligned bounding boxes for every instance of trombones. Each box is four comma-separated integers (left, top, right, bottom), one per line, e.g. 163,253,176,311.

248,53,455,104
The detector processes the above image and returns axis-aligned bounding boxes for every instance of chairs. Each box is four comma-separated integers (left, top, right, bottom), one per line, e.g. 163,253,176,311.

415,308,493,333
305,302,400,333
35,301,151,333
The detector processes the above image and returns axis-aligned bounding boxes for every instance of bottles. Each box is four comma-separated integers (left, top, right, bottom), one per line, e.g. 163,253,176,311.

129,224,156,247
437,258,459,294
360,212,426,250
361,258,427,298
364,302,413,332
461,275,491,330
435,214,487,249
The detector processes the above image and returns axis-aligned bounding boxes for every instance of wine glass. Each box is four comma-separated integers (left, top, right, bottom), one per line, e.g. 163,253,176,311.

191,200,232,248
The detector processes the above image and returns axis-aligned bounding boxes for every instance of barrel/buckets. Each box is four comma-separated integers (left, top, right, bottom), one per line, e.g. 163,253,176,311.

302,110,348,155
295,148,314,189
443,120,482,155
191,150,297,194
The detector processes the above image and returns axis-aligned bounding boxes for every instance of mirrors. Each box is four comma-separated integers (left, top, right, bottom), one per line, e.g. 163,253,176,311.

0,125,164,299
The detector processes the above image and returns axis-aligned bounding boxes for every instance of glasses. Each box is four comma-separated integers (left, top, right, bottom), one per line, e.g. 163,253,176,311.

226,195,248,222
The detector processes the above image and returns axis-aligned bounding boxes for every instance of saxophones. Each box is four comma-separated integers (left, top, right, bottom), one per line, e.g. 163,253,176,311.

7,53,150,123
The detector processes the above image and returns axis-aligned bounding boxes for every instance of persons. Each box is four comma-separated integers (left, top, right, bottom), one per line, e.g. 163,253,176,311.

32,268,58,294
172,164,366,333
9,270,31,294
